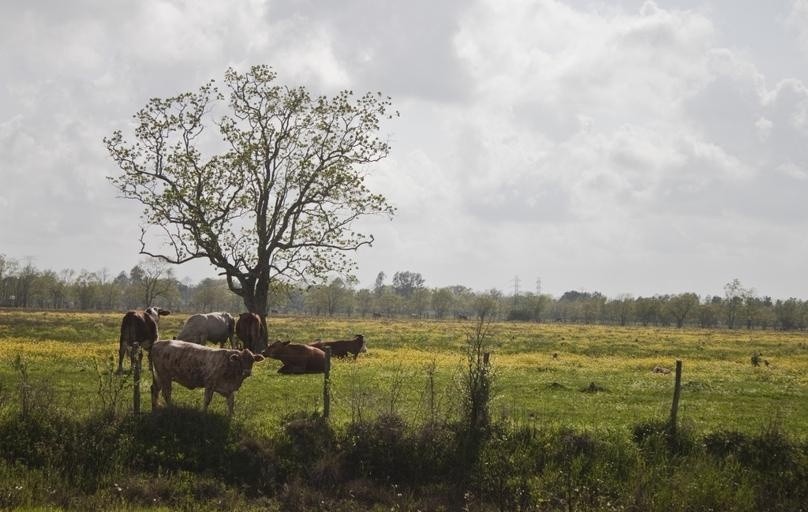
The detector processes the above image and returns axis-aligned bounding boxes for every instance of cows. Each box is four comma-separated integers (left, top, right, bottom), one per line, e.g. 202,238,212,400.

150,339,265,416
261,341,332,373
308,335,367,359
235,313,264,354
117,306,170,374
173,311,235,349
